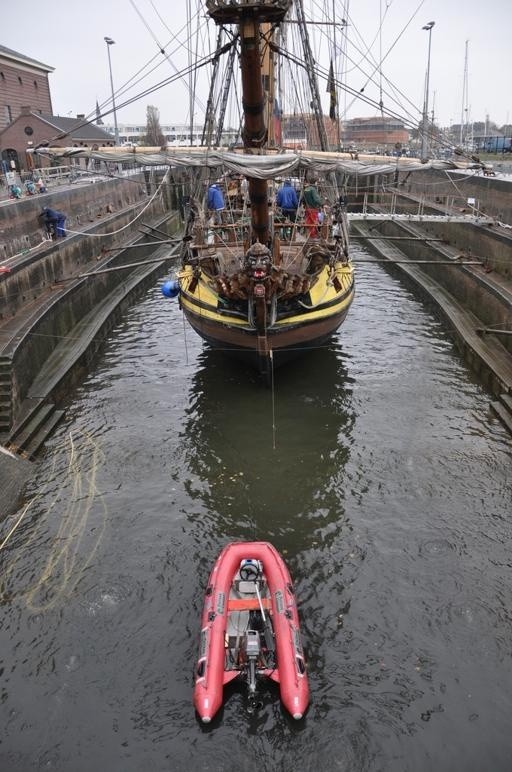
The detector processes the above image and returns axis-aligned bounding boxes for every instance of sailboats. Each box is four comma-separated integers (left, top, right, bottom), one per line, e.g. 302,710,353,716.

171,1,359,366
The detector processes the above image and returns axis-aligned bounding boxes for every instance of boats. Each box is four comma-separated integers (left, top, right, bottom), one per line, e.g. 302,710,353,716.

190,542,317,727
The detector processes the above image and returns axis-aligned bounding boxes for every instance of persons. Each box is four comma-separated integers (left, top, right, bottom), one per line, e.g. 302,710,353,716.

39,205,59,241
276,179,300,241
303,177,326,239
206,182,228,226
55,211,67,240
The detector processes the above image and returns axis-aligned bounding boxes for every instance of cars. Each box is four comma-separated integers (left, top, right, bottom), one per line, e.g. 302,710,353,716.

70,170,102,184
368,143,479,161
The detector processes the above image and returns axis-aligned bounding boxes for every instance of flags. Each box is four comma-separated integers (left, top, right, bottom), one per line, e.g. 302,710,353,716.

325,59,338,122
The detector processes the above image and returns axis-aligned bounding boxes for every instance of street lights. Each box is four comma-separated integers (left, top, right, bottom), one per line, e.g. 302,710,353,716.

421,20,435,164
103,34,128,177
